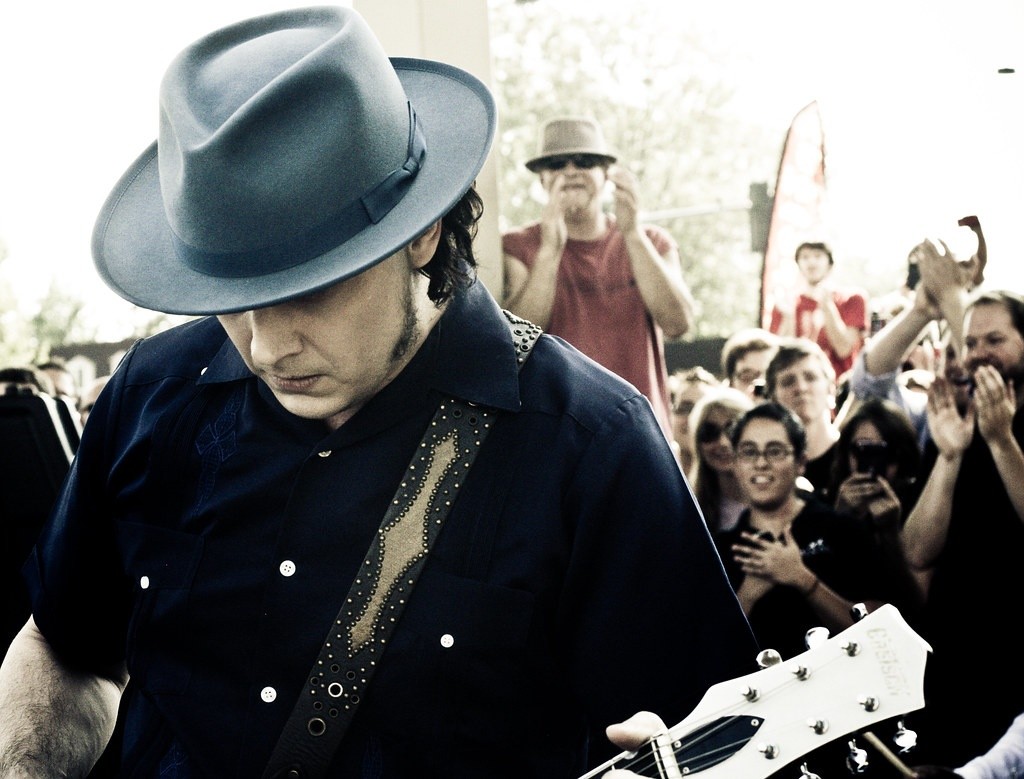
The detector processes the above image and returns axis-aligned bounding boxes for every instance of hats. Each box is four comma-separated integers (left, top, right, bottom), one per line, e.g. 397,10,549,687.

91,4,496,317
526,117,616,173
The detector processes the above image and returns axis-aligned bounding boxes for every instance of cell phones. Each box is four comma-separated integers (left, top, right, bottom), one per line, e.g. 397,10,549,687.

855,440,892,504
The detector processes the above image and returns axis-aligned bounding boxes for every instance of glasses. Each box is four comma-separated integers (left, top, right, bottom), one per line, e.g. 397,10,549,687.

734,445,797,469
671,400,696,419
698,421,740,446
544,153,597,171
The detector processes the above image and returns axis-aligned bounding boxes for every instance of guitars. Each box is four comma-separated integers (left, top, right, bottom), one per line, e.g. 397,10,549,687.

567,601,937,779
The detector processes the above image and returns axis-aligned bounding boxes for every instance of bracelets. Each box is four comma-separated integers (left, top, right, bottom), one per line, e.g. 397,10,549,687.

803,577,819,596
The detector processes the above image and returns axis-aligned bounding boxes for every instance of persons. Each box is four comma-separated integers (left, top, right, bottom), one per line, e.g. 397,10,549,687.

665,214,1024,779
0,5,807,779
502,119,696,445
0,363,113,660
